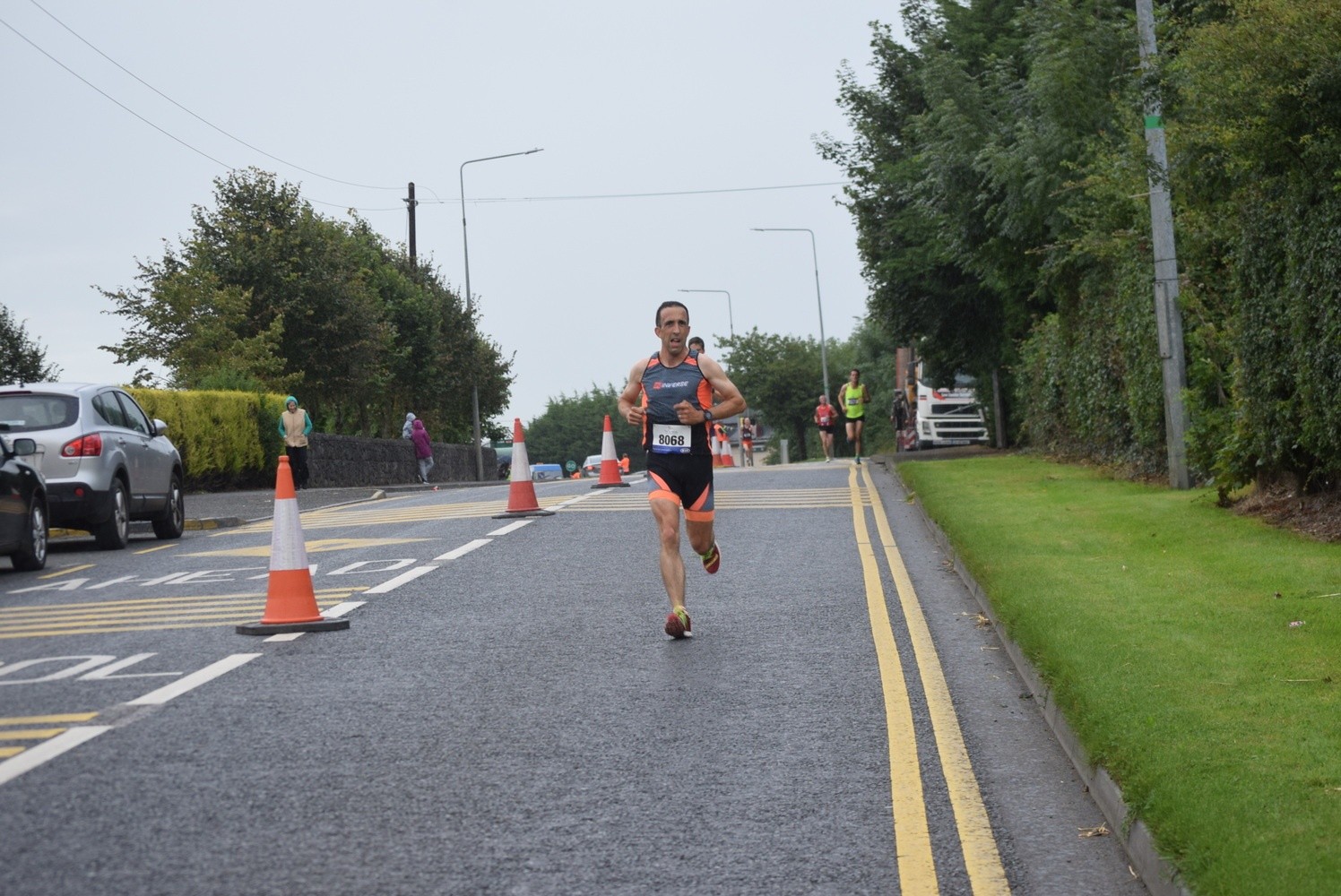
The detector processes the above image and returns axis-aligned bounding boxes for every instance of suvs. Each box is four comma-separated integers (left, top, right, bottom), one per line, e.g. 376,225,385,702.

0,380,186,549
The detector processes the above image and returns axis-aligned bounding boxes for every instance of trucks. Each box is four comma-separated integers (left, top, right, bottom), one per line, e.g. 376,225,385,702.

907,345,991,450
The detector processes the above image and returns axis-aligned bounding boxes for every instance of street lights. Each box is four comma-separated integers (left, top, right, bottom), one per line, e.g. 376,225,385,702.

677,288,746,468
459,147,545,481
749,226,836,458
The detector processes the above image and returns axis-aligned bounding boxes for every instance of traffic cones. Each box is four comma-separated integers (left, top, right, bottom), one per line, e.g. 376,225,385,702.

491,417,556,519
590,413,633,489
711,436,725,469
720,432,736,468
234,454,353,635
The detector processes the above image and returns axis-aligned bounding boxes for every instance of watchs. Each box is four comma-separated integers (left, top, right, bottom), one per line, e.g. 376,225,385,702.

702,407,713,423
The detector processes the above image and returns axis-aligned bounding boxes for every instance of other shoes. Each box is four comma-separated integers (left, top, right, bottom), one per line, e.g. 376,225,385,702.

421,481,430,483
825,458,830,463
854,456,862,465
418,473,423,483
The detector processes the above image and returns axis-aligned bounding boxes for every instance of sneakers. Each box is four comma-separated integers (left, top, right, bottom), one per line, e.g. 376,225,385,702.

664,606,693,639
701,540,721,574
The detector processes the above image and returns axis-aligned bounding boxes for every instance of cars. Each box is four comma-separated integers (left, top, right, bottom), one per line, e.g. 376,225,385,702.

579,454,625,476
0,418,51,573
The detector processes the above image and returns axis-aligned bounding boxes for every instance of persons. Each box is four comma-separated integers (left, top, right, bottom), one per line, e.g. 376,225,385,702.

619,453,630,475
616,301,754,637
814,395,839,462
412,419,433,484
403,413,416,439
278,396,313,491
838,369,871,464
